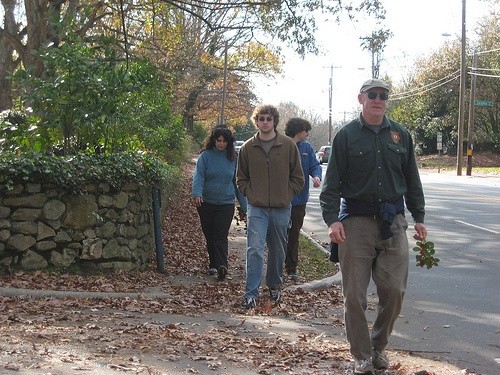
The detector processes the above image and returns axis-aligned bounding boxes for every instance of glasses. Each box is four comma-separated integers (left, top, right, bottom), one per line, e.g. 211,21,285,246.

367,92,389,100
216,138,228,142
259,117,272,121
304,128,308,132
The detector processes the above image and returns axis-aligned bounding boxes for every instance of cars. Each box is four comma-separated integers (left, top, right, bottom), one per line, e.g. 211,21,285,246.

315,145,332,164
233,140,244,152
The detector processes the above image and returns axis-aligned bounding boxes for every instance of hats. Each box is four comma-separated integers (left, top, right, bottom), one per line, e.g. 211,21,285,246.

359,79,390,92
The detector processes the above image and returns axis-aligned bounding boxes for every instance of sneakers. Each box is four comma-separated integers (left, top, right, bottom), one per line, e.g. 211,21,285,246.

371,346,390,369
353,357,374,375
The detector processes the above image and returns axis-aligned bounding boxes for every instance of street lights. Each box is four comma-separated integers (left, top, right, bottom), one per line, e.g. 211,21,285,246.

441,32,467,175
358,66,375,79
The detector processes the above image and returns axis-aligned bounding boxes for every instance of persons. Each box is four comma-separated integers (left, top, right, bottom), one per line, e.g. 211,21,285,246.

236,105,305,308
192,124,249,282
318,78,427,373
284,116,322,277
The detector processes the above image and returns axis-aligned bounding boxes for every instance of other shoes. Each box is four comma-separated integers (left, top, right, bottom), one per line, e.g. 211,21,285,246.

241,297,256,309
269,288,281,308
209,267,217,275
217,265,228,279
288,271,296,279
